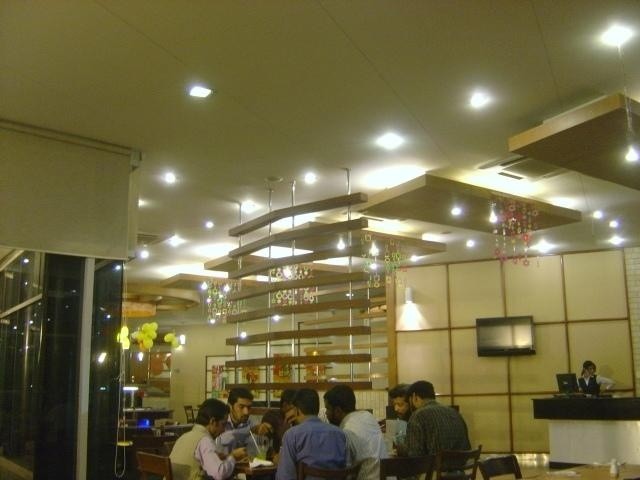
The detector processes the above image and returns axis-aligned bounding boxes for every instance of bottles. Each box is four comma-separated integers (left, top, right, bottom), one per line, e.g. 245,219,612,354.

609,458,619,478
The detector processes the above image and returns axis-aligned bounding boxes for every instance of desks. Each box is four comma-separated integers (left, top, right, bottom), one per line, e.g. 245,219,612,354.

233,462,277,480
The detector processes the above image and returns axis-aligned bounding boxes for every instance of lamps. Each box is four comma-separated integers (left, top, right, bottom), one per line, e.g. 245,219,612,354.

405,287,413,304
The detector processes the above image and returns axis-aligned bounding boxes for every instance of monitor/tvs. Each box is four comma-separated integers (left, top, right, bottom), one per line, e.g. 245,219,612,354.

476,315,536,357
556,373,579,393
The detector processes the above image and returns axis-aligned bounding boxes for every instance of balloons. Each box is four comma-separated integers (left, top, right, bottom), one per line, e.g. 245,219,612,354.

164,332,179,348
130,321,158,351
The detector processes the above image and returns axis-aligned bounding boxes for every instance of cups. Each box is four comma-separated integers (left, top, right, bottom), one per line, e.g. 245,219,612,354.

254,445,267,461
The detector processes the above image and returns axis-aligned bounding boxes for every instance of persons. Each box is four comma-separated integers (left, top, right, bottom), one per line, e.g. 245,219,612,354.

389,381,471,480
261,384,388,480
168,388,274,480
577,360,616,394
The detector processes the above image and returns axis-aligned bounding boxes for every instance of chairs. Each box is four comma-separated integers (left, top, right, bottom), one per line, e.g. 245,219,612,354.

477,455,523,479
184,405,197,424
297,459,360,480
438,444,483,479
136,450,174,480
378,456,436,479
